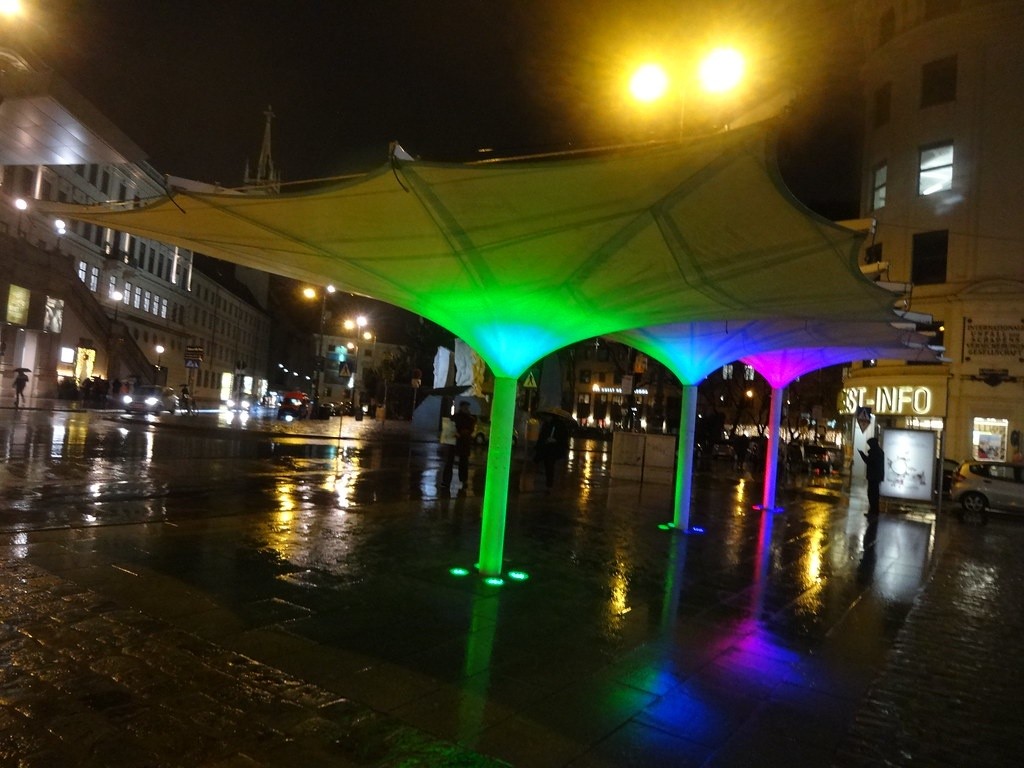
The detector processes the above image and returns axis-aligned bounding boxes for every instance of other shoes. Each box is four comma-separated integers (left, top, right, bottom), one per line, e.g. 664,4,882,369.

864,512,879,517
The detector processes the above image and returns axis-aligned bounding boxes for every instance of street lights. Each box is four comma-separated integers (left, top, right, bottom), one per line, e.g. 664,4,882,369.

636,49,740,534
113,291,122,320
53,219,66,254
15,199,26,239
306,283,333,419
344,316,372,414
155,345,164,385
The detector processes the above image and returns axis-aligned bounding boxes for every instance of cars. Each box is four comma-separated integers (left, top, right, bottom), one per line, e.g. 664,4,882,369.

228,393,258,410
277,392,308,418
712,439,734,457
124,384,178,415
470,415,518,445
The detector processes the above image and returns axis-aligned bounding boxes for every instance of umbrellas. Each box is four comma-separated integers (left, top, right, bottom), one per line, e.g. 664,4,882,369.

27,102,954,579
180,383,189,386
13,368,29,372
533,406,579,438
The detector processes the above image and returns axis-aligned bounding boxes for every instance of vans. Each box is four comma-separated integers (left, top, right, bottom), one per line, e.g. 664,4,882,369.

950,458,1024,516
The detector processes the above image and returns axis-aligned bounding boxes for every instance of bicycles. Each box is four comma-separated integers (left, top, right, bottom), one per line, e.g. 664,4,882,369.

180,396,199,418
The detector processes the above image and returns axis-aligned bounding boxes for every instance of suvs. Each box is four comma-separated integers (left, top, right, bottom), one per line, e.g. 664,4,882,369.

789,442,828,471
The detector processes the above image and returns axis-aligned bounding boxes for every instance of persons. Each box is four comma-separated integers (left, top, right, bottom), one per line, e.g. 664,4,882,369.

14,370,28,405
440,400,476,488
182,385,188,394
112,379,123,397
536,415,569,494
859,437,884,518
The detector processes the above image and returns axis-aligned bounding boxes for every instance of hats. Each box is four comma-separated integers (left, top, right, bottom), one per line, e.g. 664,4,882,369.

459,401,470,406
